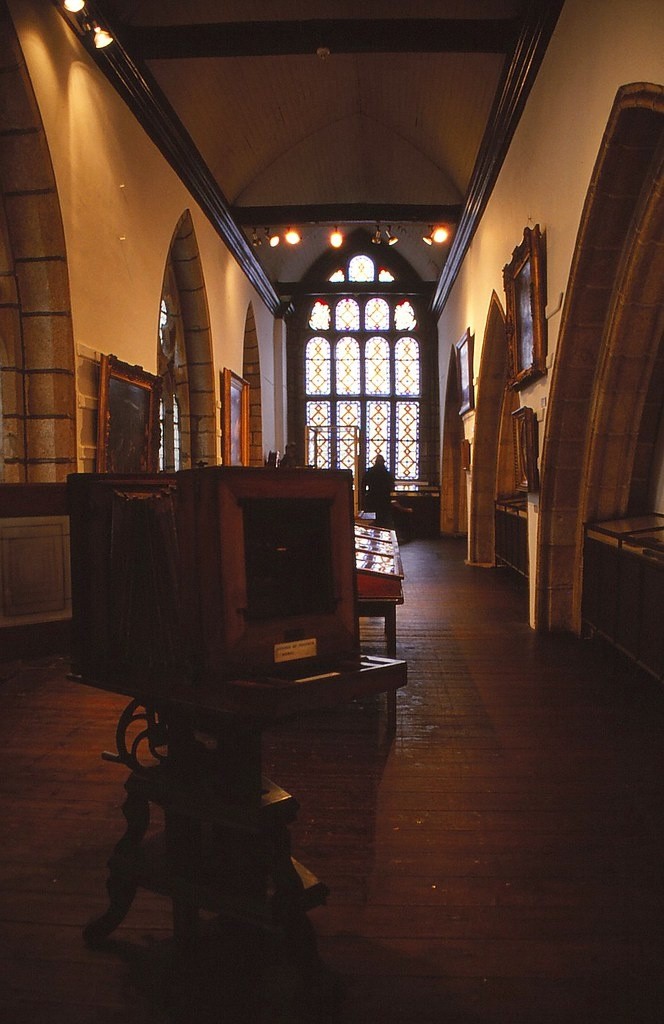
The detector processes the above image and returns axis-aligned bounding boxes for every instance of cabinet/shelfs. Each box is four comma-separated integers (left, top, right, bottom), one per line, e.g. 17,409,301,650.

582,512,664,688
494,495,529,586
352,484,440,538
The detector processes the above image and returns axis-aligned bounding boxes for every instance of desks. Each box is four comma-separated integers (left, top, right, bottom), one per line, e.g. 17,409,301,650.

353,589,403,659
59,651,407,1007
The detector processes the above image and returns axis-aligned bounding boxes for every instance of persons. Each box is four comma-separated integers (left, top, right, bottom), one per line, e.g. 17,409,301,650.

362,456,395,519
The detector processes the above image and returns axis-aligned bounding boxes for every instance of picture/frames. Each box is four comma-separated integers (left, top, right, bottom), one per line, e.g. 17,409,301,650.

222,366,250,466
95,352,162,474
454,326,474,415
511,405,539,486
501,223,547,391
460,438,468,469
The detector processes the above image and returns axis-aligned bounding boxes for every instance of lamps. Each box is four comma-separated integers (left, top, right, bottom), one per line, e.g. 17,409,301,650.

251,227,261,246
263,227,278,247
92,20,113,48
384,224,398,245
371,224,381,244
422,225,436,245
